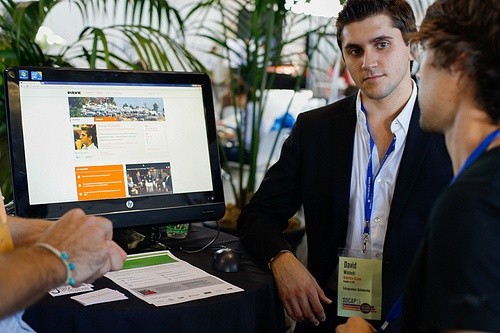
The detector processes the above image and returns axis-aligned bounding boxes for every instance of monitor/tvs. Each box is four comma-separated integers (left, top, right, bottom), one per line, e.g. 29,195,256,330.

4,65,226,253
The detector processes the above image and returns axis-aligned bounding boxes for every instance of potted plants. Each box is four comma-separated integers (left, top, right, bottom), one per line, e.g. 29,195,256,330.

179,1,341,252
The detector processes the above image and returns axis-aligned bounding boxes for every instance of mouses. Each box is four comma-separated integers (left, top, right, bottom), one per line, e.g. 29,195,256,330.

212,247,241,272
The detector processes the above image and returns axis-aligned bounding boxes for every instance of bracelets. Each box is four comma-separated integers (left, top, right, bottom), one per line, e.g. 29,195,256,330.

34,242,76,286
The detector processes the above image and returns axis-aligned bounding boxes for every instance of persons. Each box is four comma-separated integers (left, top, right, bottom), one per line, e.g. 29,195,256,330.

0,189,128,333
235,0,453,333
69,95,174,196
208,72,255,158
332,0,500,333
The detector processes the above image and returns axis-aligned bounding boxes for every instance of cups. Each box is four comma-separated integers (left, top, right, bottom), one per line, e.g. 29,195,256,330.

166,223,189,239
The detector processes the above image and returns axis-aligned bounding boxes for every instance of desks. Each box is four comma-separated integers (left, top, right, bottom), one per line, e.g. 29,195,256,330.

21,224,288,333
